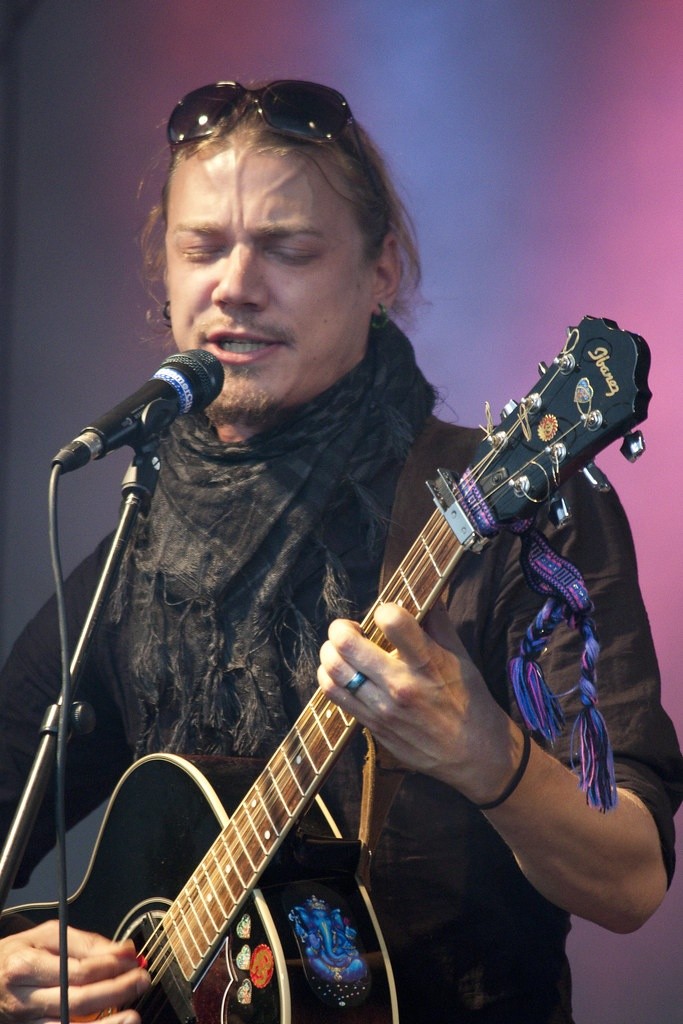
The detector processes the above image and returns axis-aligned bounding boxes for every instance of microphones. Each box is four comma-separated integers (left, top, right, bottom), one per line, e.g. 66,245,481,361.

51,349,226,475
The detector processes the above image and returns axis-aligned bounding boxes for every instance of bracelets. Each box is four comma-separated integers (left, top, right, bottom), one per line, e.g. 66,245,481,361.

468,724,532,810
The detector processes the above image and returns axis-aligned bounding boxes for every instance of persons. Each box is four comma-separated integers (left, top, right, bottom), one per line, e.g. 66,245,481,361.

2,73,683,1023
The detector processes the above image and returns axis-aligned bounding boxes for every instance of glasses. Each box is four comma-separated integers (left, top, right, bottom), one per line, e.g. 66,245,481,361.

163,79,377,188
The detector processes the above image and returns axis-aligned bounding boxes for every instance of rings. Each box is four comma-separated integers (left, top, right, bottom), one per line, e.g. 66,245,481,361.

345,671,367,696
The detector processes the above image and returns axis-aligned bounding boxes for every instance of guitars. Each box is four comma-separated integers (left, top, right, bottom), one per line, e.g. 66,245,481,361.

1,311,652,1024
